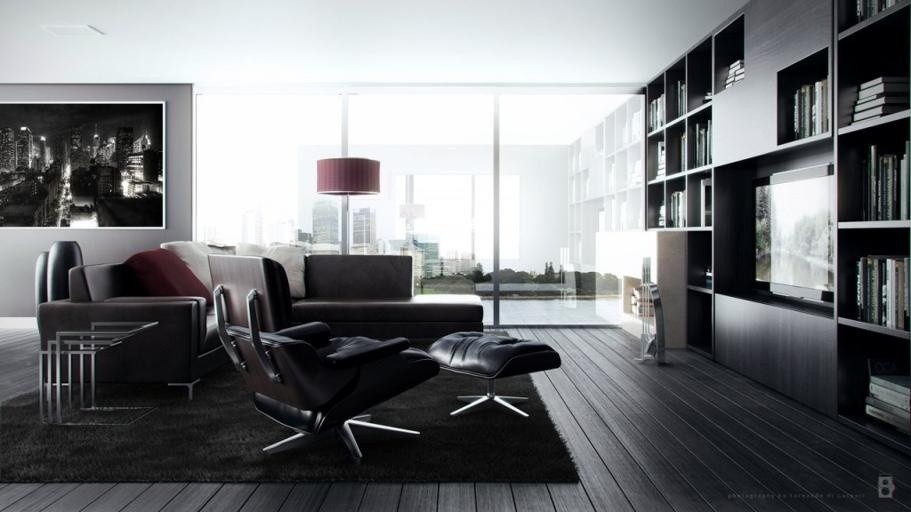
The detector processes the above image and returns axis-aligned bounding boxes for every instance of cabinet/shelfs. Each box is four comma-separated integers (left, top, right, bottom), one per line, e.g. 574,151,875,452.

566,92,648,342
642,0,911,455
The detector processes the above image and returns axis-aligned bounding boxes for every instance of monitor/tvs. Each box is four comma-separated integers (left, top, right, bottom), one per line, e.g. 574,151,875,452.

740,161,834,308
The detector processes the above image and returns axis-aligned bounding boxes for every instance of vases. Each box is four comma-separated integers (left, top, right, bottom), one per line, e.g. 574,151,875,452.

34,239,83,308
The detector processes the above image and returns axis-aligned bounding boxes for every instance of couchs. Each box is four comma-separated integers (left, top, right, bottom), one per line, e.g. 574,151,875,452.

34,253,487,400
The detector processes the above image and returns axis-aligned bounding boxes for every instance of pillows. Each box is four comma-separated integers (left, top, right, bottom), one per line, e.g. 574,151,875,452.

114,239,307,319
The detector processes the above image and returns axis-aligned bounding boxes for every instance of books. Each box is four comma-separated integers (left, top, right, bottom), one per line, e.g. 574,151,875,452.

864,372,911,434
855,0,903,25
848,75,909,124
722,58,745,91
792,74,831,140
851,138,911,332
647,81,714,229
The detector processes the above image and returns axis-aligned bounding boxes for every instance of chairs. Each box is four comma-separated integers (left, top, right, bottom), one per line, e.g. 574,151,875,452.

202,253,443,461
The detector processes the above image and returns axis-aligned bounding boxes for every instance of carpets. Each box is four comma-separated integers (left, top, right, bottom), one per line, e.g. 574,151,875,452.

0,370,583,485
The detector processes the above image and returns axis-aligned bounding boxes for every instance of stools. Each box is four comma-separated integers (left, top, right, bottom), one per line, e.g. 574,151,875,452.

429,330,562,419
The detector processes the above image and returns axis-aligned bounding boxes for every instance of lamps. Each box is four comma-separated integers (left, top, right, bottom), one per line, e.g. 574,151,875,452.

315,157,381,256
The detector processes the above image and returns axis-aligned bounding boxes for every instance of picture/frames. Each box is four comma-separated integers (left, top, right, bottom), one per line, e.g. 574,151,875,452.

0,100,169,231
